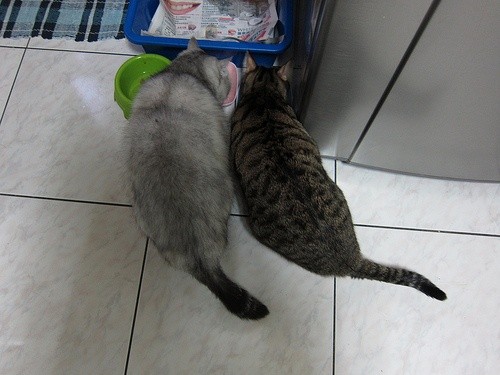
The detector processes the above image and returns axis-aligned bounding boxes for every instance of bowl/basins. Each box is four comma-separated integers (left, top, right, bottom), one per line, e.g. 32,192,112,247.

114,53,174,121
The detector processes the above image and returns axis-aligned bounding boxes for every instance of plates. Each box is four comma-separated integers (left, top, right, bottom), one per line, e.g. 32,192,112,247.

224,62,239,106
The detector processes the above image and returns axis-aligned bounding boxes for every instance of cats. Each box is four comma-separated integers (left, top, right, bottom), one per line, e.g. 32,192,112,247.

122,36,270,321
229,52,447,301
